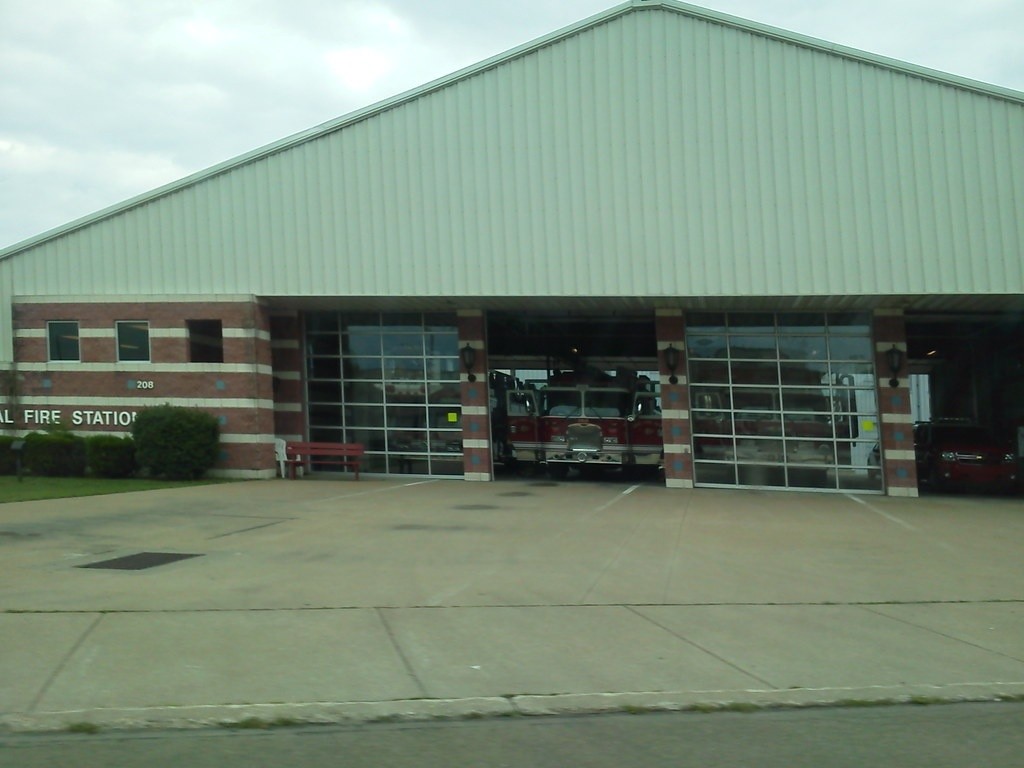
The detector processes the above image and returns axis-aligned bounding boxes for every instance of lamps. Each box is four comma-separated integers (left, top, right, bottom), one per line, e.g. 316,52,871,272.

886,344,903,388
664,343,680,384
462,342,477,383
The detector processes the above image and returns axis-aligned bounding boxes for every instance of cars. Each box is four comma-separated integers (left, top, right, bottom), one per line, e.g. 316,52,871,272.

866,417,1017,495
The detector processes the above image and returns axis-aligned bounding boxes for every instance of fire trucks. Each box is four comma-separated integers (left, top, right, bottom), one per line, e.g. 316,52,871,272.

688,347,858,486
504,364,667,483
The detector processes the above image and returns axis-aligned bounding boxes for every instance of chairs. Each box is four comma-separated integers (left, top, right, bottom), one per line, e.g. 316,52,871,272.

275,439,304,479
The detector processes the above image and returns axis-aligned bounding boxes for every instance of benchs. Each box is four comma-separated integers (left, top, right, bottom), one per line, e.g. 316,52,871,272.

284,441,364,479
